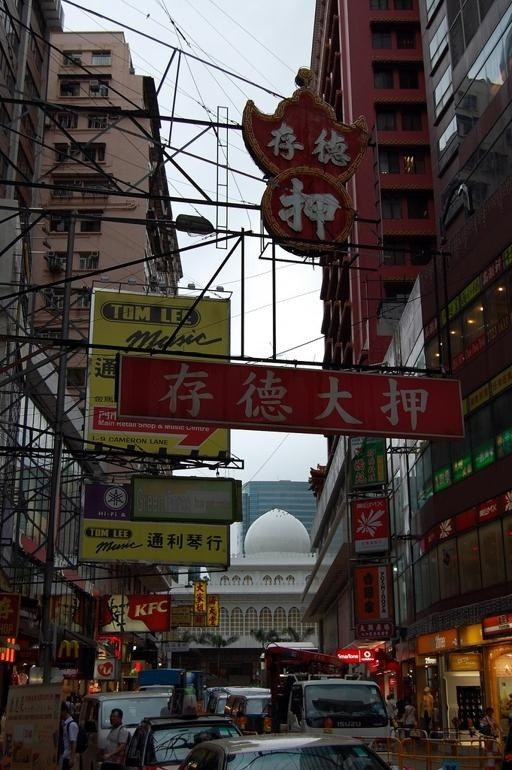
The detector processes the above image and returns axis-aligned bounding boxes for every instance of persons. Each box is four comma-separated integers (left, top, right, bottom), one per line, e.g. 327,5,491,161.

101,593,151,634
419,686,434,738
506,693,511,719
58,701,80,770
100,709,132,770
500,717,512,770
63,692,88,721
382,694,418,745
478,707,503,761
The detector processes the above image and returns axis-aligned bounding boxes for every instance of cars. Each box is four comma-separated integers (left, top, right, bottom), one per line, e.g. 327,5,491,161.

122,715,243,770
179,734,391,770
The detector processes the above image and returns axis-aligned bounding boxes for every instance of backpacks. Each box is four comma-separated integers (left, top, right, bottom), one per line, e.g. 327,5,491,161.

67,720,89,754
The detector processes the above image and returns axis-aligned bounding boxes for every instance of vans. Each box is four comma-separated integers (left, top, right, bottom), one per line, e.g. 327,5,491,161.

77,691,172,770
223,693,273,734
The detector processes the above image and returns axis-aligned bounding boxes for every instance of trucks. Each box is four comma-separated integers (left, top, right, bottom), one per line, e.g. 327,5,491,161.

202,686,270,717
136,668,202,697
277,672,397,770
